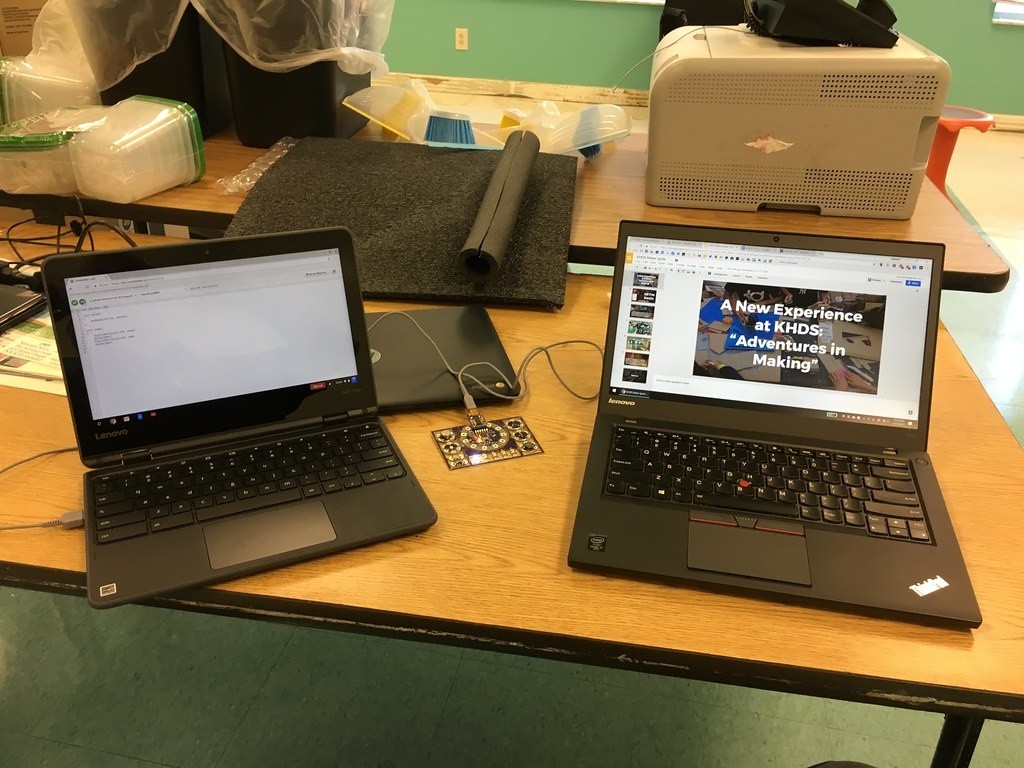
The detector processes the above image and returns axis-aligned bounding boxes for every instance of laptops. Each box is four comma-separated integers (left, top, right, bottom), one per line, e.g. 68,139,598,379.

362,306,522,415
40,226,438,613
565,220,982,634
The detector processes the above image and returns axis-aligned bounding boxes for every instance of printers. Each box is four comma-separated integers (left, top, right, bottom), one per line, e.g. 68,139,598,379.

643,20,952,221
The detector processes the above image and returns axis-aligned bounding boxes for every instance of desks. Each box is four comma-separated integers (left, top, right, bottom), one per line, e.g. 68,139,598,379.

1,78,1015,297
0,216,1023,767
